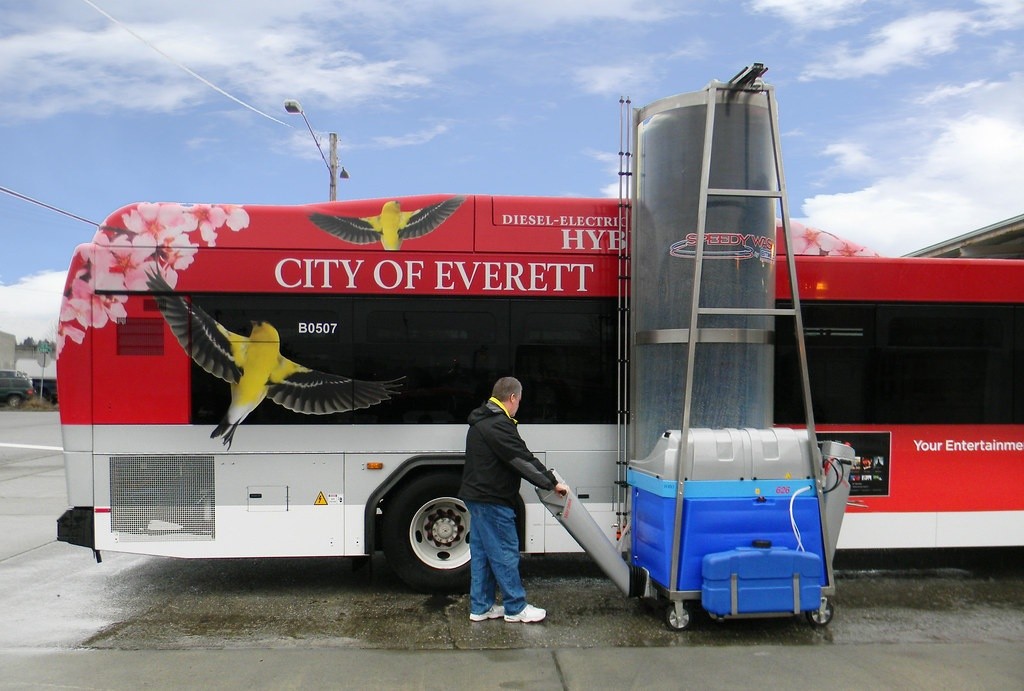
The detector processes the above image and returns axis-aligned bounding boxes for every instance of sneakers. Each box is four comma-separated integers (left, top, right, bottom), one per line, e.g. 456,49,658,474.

503,604,547,623
469,602,505,621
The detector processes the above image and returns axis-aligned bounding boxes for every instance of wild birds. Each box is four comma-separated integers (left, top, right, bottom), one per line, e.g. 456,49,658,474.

144,255,407,452
307,195,470,251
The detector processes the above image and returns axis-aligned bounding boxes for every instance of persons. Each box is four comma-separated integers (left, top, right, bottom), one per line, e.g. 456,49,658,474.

459,378,569,622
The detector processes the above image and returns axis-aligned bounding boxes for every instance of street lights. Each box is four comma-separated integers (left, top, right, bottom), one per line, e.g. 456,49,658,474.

284,98,350,203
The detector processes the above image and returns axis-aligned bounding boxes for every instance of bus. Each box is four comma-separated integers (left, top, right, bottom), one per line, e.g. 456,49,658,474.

57,192,1024,598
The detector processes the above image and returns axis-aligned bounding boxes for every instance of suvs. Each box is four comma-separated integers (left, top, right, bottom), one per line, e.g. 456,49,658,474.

0,369,35,410
